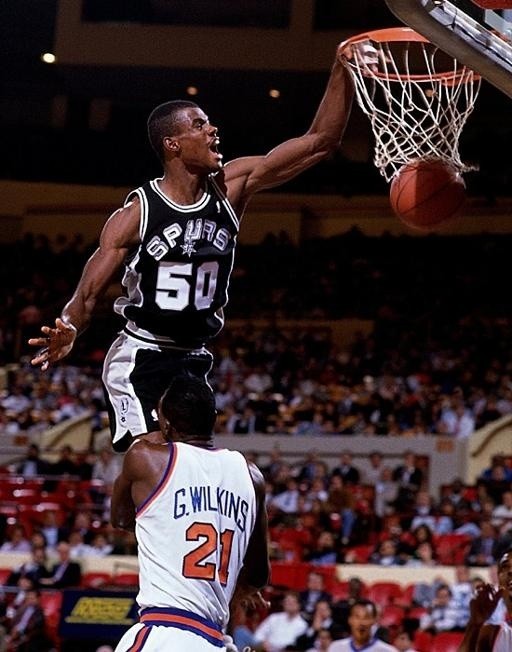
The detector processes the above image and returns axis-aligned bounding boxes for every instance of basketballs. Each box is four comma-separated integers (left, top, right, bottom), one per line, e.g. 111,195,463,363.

389,156,467,230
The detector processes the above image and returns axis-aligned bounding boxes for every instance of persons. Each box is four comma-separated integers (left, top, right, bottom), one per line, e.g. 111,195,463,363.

28,40,392,610
